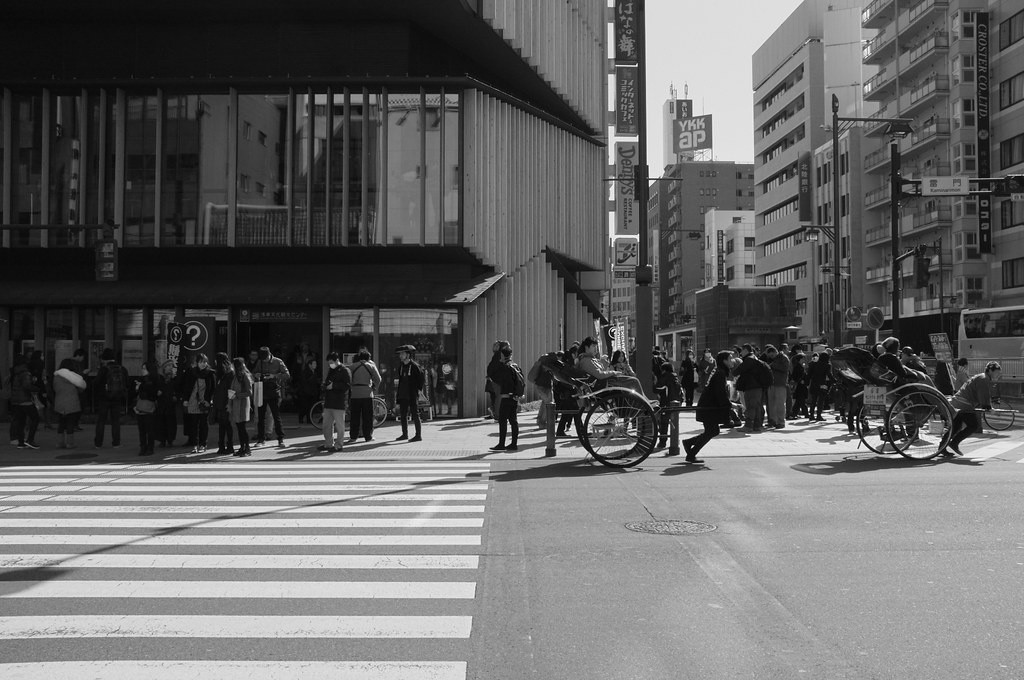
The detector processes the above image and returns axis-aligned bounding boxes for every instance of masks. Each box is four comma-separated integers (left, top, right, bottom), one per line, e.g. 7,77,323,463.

689,355,693,359
705,353,712,357
329,364,337,369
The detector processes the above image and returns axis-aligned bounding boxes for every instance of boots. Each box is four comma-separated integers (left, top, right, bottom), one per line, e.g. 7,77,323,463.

56,433,78,449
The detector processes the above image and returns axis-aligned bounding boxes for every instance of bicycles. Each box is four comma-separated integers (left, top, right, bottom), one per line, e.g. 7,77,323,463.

309,388,389,428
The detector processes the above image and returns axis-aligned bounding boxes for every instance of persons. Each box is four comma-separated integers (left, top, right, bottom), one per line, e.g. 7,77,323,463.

485,338,1003,460
7,346,424,457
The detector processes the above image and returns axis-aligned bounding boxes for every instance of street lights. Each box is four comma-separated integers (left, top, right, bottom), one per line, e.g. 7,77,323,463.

658,228,704,330
829,92,914,350
801,224,842,348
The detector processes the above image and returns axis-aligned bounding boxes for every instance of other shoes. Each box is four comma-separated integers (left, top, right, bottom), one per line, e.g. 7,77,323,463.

217,442,225,454
409,435,422,441
234,447,250,456
948,442,963,455
25,440,40,449
490,445,505,451
318,445,326,450
199,445,206,453
505,444,518,452
329,446,342,452
682,439,692,453
255,441,266,447
191,446,198,453
17,444,28,448
863,430,875,434
686,456,704,463
738,427,752,432
396,435,407,440
224,441,234,454
488,406,496,418
938,446,955,457
787,413,827,421
279,442,285,448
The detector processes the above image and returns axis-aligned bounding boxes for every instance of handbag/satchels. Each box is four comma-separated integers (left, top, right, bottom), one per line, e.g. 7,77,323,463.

254,378,263,407
134,399,154,416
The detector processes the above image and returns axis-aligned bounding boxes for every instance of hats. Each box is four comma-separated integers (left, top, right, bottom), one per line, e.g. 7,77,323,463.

902,346,913,356
395,345,415,354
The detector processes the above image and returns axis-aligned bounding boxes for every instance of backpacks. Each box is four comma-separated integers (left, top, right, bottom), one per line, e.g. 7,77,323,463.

104,365,127,400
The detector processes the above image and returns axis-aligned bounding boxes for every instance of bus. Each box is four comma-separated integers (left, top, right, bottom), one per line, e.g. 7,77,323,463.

957,305,1024,389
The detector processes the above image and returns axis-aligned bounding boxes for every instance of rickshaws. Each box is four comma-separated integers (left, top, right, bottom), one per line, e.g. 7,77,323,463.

535,352,743,467
828,345,1021,462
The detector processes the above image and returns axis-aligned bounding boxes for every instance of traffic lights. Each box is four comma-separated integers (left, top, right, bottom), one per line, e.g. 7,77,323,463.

1005,175,1024,193
913,255,931,289
